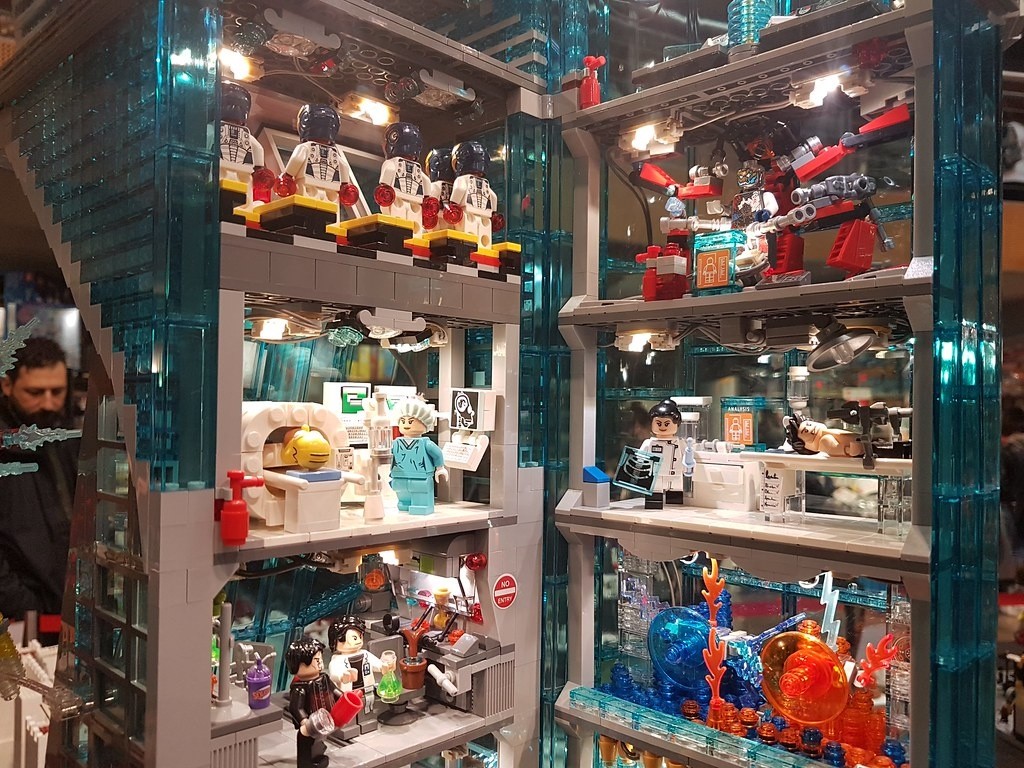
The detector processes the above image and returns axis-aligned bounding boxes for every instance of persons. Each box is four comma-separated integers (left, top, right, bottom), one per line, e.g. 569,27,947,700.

266,425,331,469
287,638,344,768
706,161,778,265
1,337,83,620
374,121,505,248
389,398,448,514
785,403,894,457
640,400,696,510
205,81,275,212
273,102,359,227
328,615,396,739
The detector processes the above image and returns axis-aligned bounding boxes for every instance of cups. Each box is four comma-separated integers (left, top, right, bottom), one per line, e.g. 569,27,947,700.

330,689,363,727
246,659,271,709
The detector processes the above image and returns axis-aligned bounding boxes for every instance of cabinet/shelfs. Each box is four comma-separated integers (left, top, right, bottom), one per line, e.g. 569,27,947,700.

0,0,1003,768
